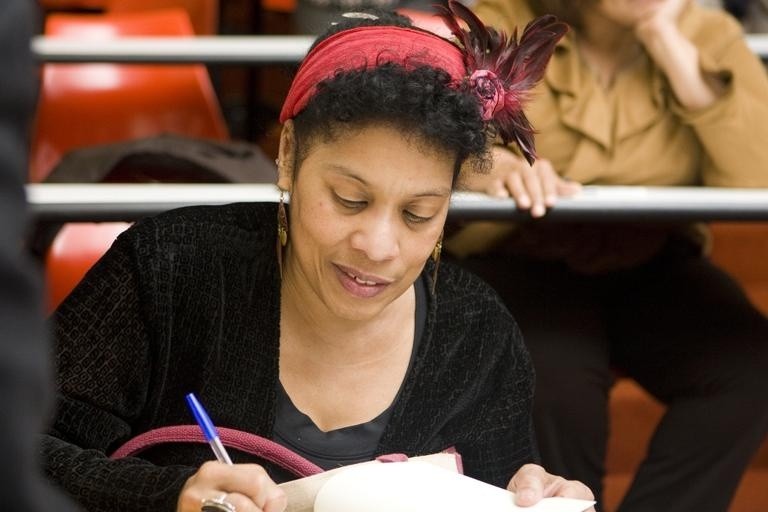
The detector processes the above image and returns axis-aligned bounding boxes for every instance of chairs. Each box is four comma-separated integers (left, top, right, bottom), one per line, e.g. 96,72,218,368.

32,13,232,330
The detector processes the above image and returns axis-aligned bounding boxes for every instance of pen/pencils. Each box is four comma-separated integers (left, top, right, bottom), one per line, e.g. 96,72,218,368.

184,392,233,466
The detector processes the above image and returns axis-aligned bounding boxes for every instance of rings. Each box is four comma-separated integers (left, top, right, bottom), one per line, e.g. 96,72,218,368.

199,492,235,511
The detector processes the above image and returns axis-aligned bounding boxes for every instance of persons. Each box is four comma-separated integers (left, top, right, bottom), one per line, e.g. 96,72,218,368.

24,6,596,511
448,0,766,511
1,1,73,510
